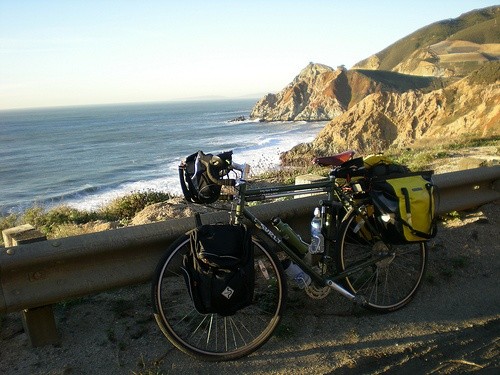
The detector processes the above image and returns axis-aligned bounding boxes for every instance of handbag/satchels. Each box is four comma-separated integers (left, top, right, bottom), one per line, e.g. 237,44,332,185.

180,150,222,204
368,162,441,246
329,151,412,245
180,223,254,318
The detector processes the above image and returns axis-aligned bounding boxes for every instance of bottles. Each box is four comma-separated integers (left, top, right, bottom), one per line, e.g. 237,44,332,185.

281,259,312,289
320,212,334,261
270,216,309,255
309,207,325,255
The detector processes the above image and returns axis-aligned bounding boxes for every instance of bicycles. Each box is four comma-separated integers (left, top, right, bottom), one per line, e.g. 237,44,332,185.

150,150,430,363
